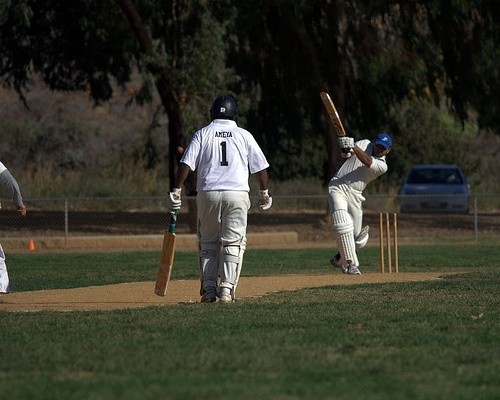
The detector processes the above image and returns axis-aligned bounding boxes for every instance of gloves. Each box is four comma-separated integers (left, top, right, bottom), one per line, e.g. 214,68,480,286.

340,150,353,158
338,136,355,149
167,188,182,215
258,189,273,210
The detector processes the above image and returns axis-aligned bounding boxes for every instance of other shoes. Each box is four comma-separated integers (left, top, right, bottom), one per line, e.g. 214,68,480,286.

200,293,216,302
219,292,235,303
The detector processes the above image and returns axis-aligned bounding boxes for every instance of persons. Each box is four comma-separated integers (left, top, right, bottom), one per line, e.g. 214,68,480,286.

328,134,393,276
167,95,273,303
0,161,26,294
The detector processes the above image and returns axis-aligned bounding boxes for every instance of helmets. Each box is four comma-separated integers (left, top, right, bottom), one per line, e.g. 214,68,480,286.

210,95,238,118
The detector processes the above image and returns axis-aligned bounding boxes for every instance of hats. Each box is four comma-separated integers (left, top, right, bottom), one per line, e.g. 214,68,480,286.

373,132,392,149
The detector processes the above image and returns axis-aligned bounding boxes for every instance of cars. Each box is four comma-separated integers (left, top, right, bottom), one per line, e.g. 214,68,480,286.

401,164,469,214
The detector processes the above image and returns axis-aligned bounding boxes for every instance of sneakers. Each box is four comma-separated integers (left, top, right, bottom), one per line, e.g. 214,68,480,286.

330,257,342,269
342,265,361,274
